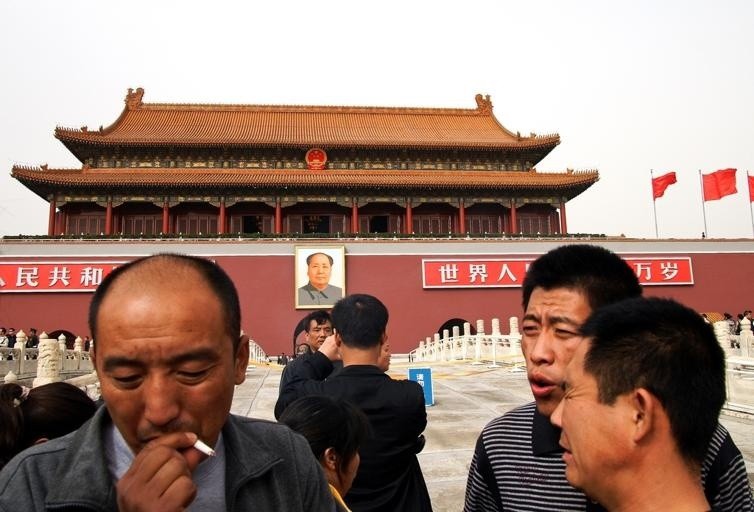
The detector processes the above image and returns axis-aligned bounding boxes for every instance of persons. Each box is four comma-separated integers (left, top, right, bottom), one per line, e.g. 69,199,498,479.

278,394,369,511
265,352,291,365
376,335,390,373
274,294,433,512
0,381,99,471
6,328,16,359
702,310,754,347
463,244,754,512
278,310,342,397
550,295,725,510
84,335,89,351
0,327,8,361
0,253,337,510
25,328,37,359
297,253,341,306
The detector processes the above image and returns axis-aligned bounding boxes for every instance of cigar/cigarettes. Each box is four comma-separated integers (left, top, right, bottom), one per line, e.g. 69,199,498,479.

191,438,216,459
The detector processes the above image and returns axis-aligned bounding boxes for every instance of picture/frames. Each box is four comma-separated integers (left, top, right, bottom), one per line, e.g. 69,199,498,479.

294,245,346,310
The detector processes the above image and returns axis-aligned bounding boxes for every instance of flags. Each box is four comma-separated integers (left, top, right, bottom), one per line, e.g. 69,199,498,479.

652,172,677,200
748,175,754,203
702,169,738,202
